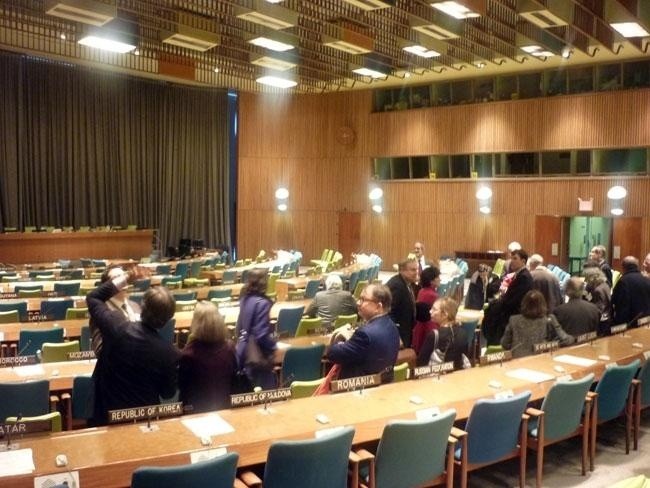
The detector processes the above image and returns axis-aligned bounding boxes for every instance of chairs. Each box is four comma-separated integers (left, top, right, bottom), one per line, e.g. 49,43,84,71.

525,357,650,487
393,362,409,382
1,302,29,322
61,377,107,430
459,318,478,342
294,317,322,337
352,409,460,488
239,425,361,488
65,307,90,320
450,391,532,488
19,328,65,356
40,300,74,320
5,411,62,436
2,250,622,305
334,313,358,332
80,326,90,351
290,378,325,400
277,306,305,337
483,346,502,355
1,310,20,323
131,452,249,488
41,339,80,362
283,344,326,389
0,379,60,439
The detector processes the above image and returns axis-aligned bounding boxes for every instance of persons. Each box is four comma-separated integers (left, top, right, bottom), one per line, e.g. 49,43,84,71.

301,243,468,388
465,242,650,360
209,243,230,266
233,262,284,391
91,263,142,358
180,297,238,415
83,260,181,426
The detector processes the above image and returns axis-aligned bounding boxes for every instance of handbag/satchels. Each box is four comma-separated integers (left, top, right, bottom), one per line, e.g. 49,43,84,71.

245,335,276,366
